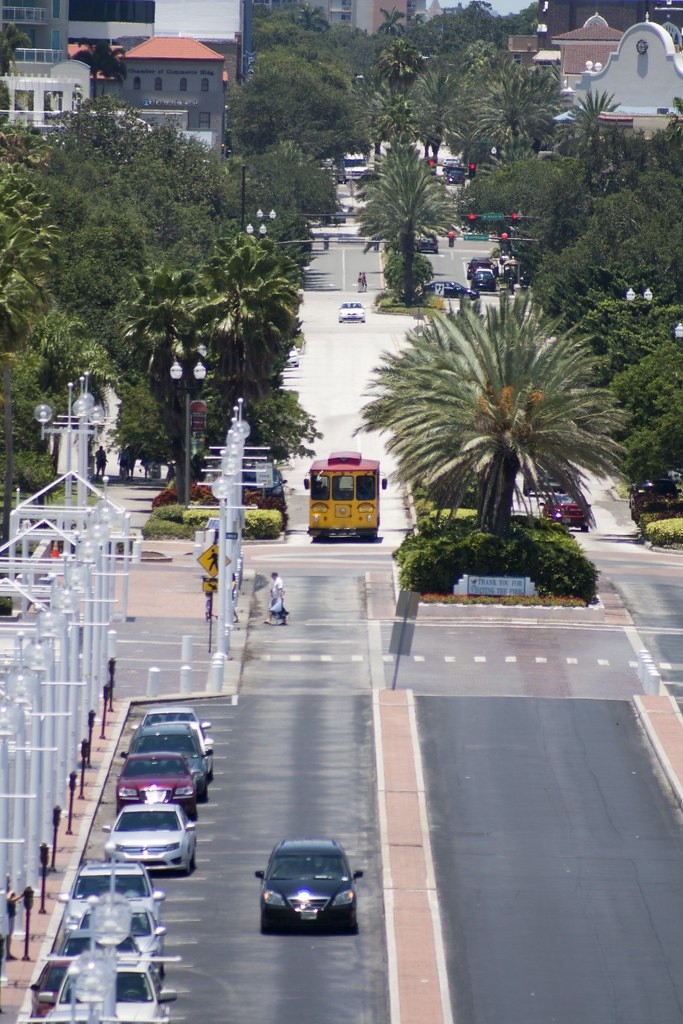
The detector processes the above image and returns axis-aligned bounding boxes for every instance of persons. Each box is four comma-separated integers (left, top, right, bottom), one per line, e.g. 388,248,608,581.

96,446,106,476
204,592,210,622
165,461,176,479
118,446,136,481
232,573,239,623
357,272,367,293
263,572,287,626
140,459,150,479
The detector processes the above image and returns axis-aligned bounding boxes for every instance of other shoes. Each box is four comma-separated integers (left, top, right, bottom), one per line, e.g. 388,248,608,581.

279,621,287,626
234,620,239,623
263,620,271,625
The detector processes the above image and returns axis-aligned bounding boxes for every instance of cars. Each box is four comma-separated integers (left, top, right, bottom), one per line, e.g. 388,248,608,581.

31,959,72,1017
628,478,679,521
337,301,366,323
115,751,202,820
416,236,438,253
101,802,198,876
424,280,478,300
60,905,167,977
522,459,592,531
443,156,465,186
466,257,498,292
255,834,365,934
128,706,214,803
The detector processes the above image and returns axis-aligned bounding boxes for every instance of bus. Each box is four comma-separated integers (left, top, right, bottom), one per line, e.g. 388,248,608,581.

303,451,387,542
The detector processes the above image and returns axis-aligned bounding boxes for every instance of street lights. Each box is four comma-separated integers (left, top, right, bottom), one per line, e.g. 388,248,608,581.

170,361,205,508
212,421,250,661
35,391,106,628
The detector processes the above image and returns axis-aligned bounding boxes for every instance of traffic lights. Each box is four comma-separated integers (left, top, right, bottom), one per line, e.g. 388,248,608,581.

469,213,474,228
502,233,508,248
448,232,455,248
428,160,435,176
469,163,475,179
513,212,518,228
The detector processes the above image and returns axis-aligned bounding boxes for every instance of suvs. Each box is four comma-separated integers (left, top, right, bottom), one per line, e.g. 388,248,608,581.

342,155,370,180
58,859,166,934
42,950,177,1023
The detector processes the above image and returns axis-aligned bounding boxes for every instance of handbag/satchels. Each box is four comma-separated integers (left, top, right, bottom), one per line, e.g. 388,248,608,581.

269,596,284,613
